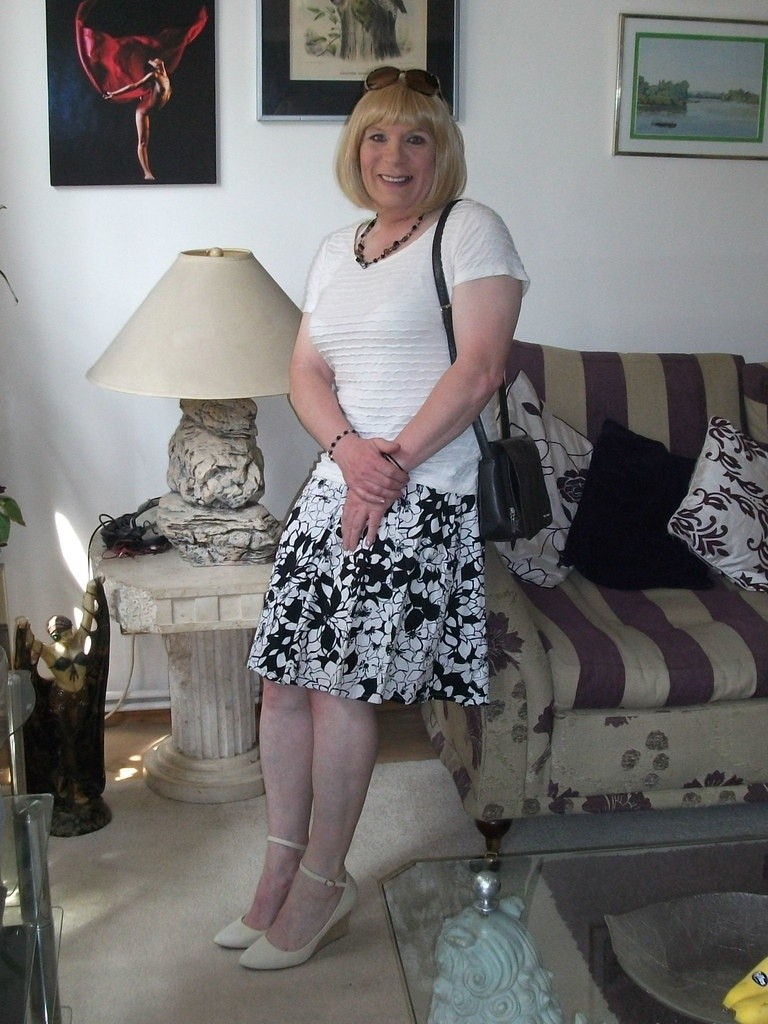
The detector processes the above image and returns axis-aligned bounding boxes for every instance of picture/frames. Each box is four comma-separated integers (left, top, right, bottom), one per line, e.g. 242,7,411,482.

254,0,460,123
613,12,768,160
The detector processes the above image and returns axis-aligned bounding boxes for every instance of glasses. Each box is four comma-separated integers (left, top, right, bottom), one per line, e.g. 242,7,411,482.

343,65,449,126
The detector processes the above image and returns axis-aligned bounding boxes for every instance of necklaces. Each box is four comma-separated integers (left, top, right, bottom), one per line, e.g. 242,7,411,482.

356,211,424,268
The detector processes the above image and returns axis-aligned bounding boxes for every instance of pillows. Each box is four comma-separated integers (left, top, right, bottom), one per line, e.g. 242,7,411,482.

667,413,768,590
557,416,713,591
486,369,595,591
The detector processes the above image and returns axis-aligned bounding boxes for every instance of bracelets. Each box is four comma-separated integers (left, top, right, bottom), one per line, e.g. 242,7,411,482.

329,429,360,461
381,452,409,474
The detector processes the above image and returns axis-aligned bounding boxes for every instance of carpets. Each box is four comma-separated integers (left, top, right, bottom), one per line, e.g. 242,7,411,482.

0,760,768,1024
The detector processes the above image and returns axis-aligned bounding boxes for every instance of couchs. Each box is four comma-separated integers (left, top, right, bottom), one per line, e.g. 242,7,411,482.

418,339,768,853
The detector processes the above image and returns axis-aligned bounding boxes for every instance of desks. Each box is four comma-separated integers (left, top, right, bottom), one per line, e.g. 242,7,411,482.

90,531,274,802
377,841,768,1024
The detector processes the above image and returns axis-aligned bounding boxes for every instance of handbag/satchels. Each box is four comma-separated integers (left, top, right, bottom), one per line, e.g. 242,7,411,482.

476,433,553,542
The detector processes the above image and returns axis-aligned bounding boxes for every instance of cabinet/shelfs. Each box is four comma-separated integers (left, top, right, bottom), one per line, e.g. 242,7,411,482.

0,645,62,1024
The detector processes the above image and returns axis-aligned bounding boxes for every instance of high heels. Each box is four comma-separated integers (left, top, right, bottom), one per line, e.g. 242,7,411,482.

212,836,307,948
238,857,358,969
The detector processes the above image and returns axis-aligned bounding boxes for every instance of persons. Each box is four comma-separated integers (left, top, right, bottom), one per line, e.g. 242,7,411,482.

103,58,172,180
15,575,105,803
213,66,531,972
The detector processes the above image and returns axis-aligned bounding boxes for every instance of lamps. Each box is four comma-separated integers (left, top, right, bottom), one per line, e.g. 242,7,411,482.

84,247,303,568
